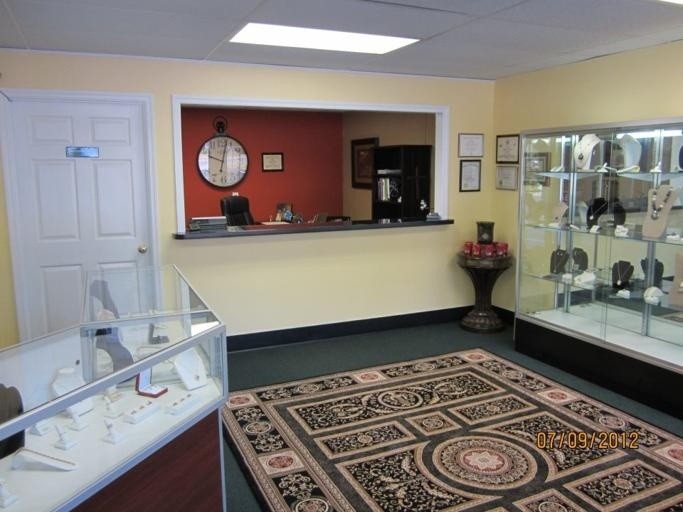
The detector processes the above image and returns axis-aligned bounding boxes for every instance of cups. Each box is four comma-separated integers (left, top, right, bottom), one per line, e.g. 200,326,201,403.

472,244,481,256
496,244,504,255
485,245,493,256
464,241,473,255
503,243,509,254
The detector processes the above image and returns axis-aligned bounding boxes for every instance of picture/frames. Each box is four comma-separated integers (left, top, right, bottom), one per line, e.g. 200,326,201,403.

494,133,552,191
458,133,485,193
350,138,379,190
262,152,283,172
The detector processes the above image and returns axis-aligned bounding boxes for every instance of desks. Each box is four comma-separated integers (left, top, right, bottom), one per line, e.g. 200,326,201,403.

458,251,513,334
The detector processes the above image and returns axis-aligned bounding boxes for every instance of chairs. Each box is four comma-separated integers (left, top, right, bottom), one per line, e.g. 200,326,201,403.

220,196,254,226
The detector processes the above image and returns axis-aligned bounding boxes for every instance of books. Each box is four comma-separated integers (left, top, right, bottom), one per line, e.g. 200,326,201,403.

376,217,401,224
188,215,228,231
377,176,401,203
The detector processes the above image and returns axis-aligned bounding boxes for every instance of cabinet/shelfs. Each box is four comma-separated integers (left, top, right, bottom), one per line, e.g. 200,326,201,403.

370,143,431,223
513,120,683,421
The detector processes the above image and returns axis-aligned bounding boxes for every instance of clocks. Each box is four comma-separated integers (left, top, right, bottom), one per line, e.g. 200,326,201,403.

195,134,250,189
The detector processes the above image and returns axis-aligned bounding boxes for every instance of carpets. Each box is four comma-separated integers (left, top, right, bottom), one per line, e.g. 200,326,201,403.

219,344,683,512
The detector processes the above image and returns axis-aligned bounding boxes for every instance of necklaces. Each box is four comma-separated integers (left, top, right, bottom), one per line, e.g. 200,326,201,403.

551,204,566,221
553,251,567,273
651,189,671,220
179,355,200,380
617,262,631,287
678,280,683,293
53,376,82,390
588,202,609,221
576,139,594,159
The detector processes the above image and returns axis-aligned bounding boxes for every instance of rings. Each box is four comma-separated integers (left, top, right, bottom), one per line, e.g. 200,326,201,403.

179,398,185,402
186,393,192,398
58,431,64,438
104,399,112,405
68,411,76,418
130,410,136,415
671,232,676,236
173,402,179,406
137,406,143,409
145,401,152,406
106,423,113,429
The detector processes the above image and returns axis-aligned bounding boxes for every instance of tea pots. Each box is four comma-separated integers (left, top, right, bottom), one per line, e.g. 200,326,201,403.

378,178,394,201
